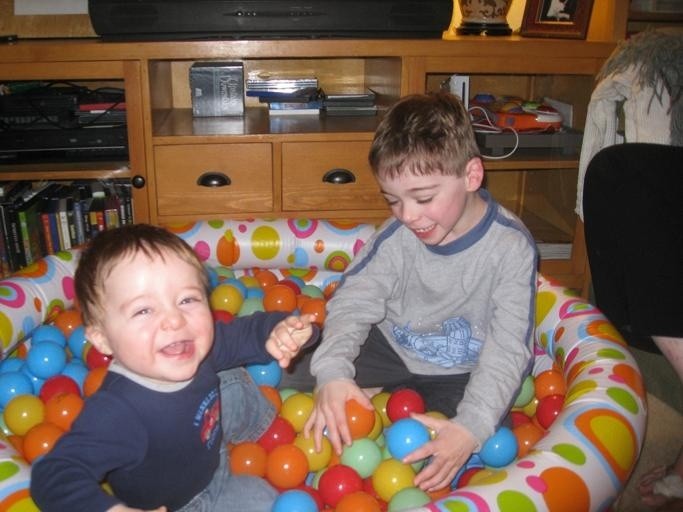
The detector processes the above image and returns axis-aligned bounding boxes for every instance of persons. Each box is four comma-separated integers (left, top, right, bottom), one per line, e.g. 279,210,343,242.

278,96,537,493
25,223,316,512
582,143,680,510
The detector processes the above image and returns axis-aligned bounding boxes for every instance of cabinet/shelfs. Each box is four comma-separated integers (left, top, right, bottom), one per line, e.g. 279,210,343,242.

133,40,426,227
0,37,152,284
398,41,624,305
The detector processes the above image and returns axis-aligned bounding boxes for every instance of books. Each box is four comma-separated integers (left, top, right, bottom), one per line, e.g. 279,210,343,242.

243,68,378,119
0,179,133,277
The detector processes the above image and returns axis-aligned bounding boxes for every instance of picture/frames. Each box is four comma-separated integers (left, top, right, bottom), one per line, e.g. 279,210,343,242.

520,0,599,39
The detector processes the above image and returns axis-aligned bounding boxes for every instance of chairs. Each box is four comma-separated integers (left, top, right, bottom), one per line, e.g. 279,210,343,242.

580,139,682,388
